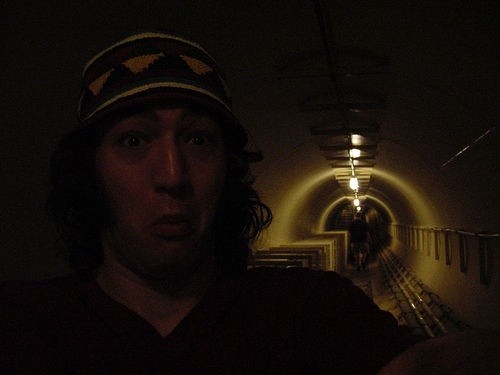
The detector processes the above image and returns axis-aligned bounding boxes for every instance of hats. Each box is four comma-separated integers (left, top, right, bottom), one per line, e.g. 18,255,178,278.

75,30,236,130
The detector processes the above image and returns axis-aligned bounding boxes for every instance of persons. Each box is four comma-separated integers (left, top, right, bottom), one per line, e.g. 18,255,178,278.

0,24,500,374
349,212,371,270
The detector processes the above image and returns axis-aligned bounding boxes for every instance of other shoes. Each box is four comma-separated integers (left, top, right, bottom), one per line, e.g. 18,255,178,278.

362,263,365,269
357,269,360,271
